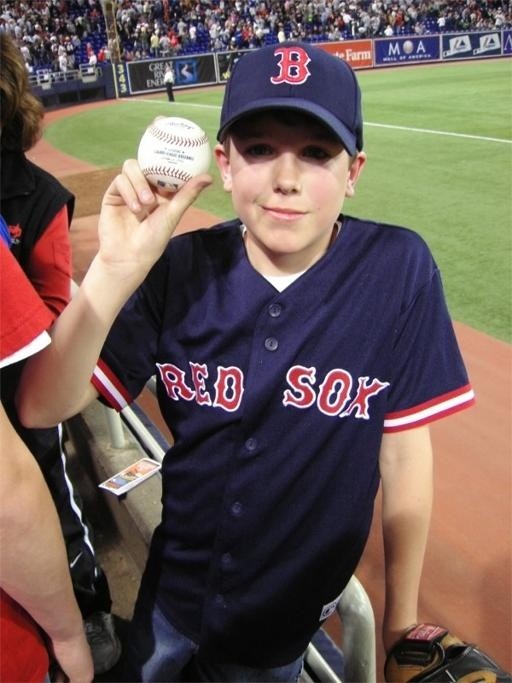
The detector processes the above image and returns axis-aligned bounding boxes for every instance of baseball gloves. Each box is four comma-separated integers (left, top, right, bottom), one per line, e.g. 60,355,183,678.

382,623,510,683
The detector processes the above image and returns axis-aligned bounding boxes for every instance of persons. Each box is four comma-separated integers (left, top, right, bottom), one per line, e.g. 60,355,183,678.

163,63,177,102
10,39,510,681
0,206,96,682
1,1,512,85
1,26,122,675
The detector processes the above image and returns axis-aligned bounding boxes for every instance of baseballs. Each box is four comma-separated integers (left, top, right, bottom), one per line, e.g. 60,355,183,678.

138,116,208,196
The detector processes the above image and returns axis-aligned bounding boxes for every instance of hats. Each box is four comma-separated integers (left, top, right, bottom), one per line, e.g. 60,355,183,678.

215,40,364,157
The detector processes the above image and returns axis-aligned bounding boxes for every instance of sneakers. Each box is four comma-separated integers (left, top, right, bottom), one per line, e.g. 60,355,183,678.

83,610,123,674
